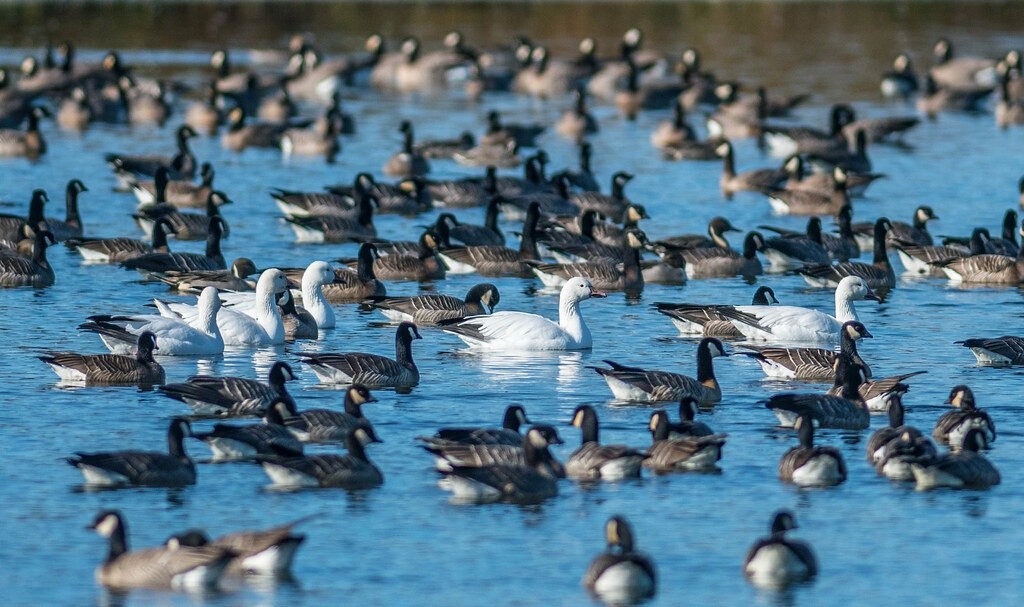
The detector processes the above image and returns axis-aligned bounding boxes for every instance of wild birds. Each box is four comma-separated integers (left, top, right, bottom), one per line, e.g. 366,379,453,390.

0,29,1024,607
